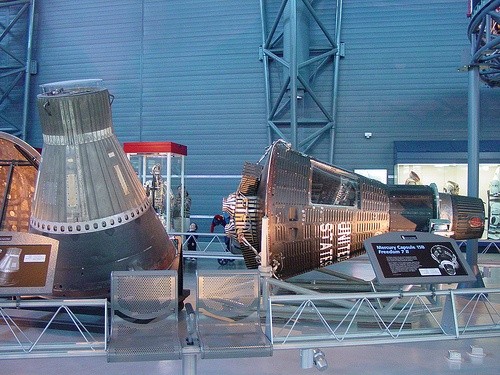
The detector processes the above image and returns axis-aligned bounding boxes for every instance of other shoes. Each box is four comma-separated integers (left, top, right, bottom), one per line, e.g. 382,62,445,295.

187,257,190,260
192,258,196,261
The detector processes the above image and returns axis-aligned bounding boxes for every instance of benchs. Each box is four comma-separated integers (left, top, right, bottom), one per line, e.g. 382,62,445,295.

106,270,274,375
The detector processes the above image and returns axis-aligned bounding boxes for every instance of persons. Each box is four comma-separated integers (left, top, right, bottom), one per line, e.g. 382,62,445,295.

172,185,192,242
186,222,198,260
